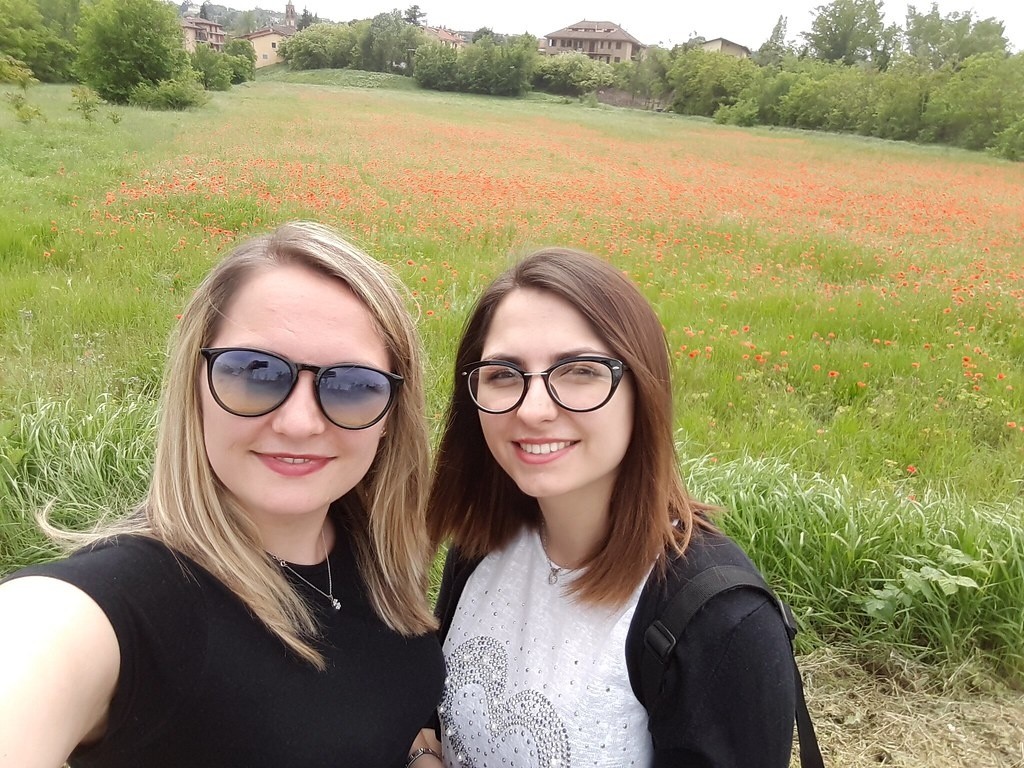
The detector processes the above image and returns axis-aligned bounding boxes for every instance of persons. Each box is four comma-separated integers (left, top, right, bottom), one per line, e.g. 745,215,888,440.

426,249,797,768
0,224,445,768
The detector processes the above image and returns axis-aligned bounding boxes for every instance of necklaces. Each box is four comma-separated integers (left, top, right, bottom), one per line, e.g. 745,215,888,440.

266,527,342,610
540,517,586,586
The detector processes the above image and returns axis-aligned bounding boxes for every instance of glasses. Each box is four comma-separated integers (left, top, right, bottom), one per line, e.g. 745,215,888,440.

200,347,405,430
461,356,632,414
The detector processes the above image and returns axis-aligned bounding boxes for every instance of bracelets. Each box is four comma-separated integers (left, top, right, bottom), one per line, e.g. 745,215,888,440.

405,748,443,767
